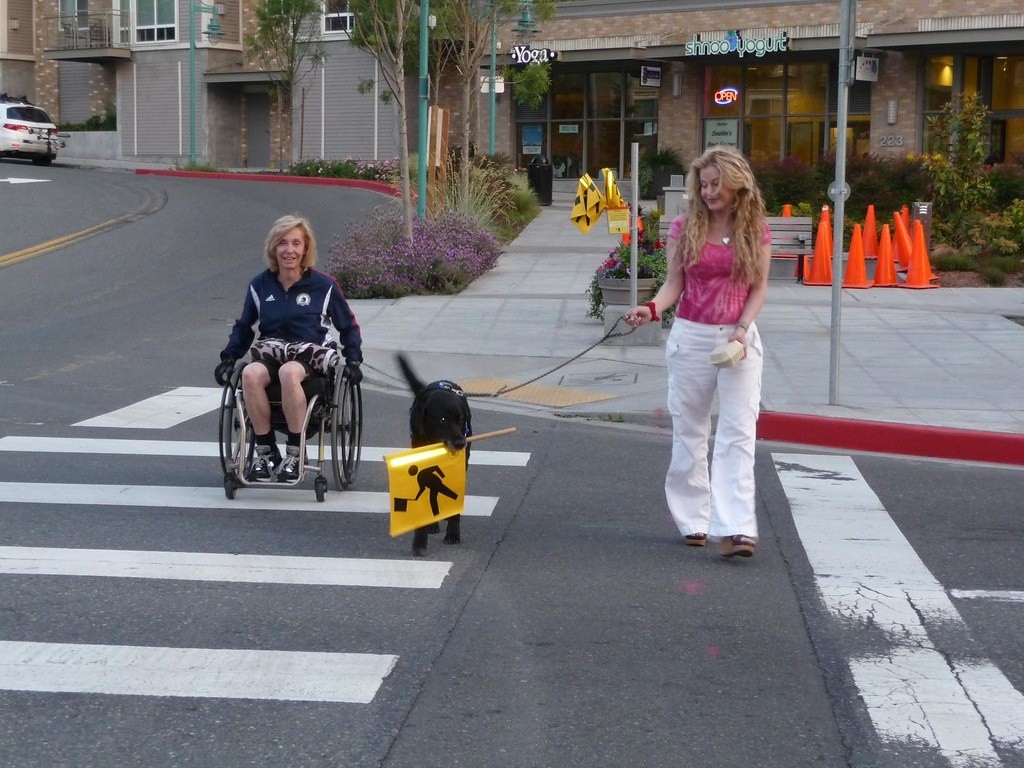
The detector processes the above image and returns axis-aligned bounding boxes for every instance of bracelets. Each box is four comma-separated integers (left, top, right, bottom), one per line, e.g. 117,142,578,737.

735,320,749,332
642,302,660,322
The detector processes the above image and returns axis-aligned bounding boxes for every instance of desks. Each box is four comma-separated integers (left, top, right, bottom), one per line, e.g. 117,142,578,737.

90,25,110,48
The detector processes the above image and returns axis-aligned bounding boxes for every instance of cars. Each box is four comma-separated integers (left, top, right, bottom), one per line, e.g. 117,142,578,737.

0,101,58,165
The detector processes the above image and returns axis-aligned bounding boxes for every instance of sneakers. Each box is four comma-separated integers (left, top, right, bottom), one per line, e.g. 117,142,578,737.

271,446,310,483
249,445,284,482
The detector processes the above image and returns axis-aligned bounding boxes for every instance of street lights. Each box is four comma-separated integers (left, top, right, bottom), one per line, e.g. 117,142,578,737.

490,3,544,156
189,5,227,163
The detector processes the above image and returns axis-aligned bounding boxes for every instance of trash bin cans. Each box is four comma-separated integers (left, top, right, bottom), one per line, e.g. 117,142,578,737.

528,155,553,205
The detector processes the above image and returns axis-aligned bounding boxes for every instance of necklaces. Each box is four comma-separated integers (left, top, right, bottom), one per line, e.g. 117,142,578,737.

709,212,734,245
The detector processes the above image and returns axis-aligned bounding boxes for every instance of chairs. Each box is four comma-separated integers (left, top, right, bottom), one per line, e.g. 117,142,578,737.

62,22,87,49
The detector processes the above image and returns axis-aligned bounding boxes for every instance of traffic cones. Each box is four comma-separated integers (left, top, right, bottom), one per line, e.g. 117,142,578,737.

811,205,834,258
770,204,800,258
802,221,832,286
793,255,810,278
891,205,939,281
899,224,940,289
861,205,879,259
872,224,901,288
842,223,875,288
623,203,645,243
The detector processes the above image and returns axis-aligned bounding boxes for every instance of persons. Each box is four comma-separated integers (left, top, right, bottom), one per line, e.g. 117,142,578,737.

215,215,363,482
621,143,770,558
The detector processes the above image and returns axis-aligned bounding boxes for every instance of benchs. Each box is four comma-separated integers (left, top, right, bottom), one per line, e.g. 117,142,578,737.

657,215,812,281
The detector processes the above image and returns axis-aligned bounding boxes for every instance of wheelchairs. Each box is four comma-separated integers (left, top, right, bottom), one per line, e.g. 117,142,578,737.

219,342,362,503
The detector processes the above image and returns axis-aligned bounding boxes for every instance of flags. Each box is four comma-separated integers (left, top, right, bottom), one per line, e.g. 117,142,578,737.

569,169,626,234
384,442,467,537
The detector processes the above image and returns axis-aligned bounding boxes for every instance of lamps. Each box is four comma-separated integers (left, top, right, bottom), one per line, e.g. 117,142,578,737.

672,74,682,97
887,99,898,125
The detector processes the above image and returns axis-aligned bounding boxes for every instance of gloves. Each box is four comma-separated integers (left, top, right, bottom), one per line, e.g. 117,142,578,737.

215,357,236,386
342,360,363,385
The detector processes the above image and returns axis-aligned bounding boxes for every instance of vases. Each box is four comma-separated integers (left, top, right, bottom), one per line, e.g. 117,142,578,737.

596,275,662,304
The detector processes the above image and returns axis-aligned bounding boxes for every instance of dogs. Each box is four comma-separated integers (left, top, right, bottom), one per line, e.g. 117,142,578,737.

395,354,473,556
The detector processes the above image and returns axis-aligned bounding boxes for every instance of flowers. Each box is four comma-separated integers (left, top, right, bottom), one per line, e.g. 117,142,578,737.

585,242,673,323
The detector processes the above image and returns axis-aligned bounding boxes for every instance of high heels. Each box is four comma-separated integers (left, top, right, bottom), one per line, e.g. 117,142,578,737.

719,535,755,558
685,533,706,546
